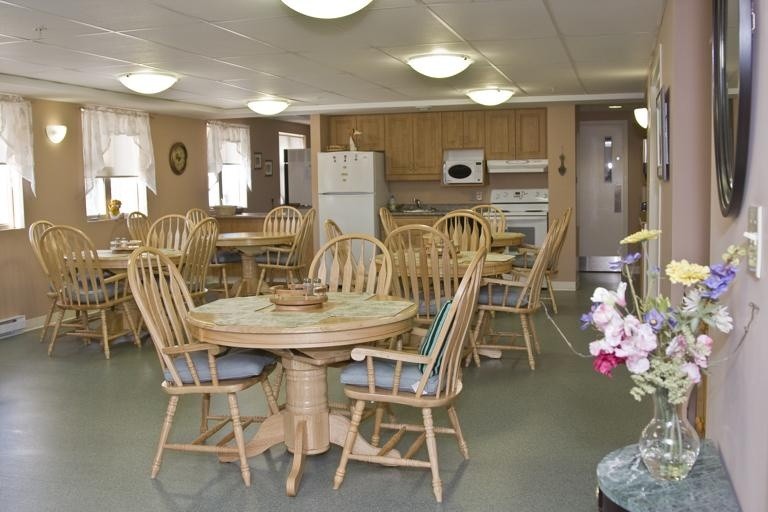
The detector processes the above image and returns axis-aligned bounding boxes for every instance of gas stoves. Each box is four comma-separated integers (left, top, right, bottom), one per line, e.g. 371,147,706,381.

490,188,549,216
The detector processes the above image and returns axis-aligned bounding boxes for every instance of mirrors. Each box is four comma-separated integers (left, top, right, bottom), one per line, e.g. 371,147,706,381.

712,0,756,217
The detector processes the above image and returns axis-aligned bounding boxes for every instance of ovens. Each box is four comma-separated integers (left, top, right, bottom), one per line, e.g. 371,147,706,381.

490,216,548,289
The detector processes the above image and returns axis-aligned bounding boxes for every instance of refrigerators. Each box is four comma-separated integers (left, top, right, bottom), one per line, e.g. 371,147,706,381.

317,151,385,286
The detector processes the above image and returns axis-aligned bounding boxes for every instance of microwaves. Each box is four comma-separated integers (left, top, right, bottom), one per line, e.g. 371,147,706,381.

443,160,483,184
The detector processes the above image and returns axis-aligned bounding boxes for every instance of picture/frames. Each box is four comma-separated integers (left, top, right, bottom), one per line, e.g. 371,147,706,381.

253,152,263,169
264,160,273,176
655,87,669,181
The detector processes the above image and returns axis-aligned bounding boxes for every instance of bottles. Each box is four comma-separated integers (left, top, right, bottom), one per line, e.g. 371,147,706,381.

115,238,121,248
303,278,313,296
314,279,321,295
389,195,396,212
121,237,128,248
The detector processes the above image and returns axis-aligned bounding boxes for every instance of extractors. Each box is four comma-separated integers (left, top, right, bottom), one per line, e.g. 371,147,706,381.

487,159,548,174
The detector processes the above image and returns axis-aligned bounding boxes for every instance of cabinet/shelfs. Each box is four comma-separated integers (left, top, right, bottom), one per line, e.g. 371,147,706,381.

383,112,444,181
329,113,384,152
484,108,545,159
441,111,484,149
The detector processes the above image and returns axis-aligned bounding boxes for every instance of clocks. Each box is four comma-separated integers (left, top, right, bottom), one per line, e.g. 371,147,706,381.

169,142,187,175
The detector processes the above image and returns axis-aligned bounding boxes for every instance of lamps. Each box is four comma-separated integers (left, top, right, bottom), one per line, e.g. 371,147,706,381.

281,0,373,20
634,107,648,129
116,72,179,94
246,99,290,115
46,125,67,144
465,88,514,106
407,54,472,78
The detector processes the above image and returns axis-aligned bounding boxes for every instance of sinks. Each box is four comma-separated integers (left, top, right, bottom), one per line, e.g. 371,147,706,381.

401,209,434,213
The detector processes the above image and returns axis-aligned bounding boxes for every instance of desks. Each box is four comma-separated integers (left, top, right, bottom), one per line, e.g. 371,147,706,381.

596,439,742,512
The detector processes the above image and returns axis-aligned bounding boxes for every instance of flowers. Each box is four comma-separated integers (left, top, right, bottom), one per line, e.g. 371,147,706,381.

521,229,760,405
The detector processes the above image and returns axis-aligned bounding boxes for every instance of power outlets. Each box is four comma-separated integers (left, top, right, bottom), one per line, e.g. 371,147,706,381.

747,206,761,279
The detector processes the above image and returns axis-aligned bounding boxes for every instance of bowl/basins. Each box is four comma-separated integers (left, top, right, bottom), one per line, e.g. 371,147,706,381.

213,206,237,216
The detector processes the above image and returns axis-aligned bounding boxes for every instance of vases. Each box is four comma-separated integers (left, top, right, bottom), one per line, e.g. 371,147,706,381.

639,383,701,481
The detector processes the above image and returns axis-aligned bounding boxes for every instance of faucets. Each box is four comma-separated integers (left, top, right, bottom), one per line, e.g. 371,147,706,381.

412,196,421,208
